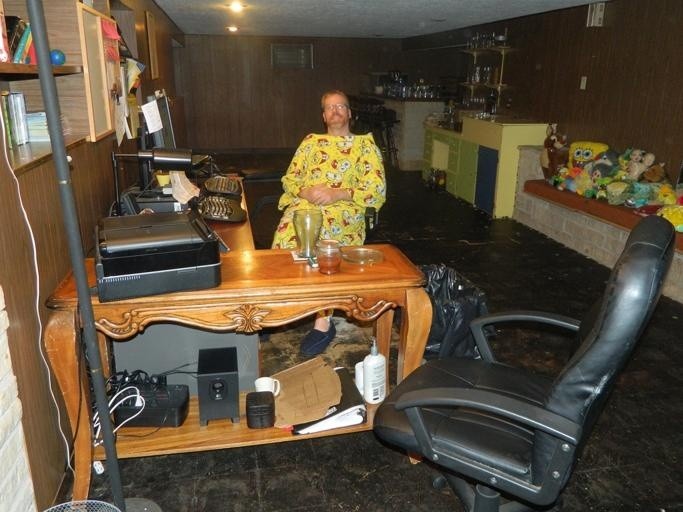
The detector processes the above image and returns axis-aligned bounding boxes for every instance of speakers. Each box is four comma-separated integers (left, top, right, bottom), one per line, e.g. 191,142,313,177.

198,347,240,426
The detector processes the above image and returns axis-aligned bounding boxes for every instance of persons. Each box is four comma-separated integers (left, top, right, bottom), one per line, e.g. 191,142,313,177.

270,89,389,360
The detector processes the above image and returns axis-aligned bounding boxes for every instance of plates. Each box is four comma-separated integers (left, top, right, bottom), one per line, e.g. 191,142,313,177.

342,248,385,265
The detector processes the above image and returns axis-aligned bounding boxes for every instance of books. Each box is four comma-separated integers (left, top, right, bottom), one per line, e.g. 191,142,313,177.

0,0,72,149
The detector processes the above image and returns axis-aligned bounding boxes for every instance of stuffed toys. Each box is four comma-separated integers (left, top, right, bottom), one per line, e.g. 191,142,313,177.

539,124,683,233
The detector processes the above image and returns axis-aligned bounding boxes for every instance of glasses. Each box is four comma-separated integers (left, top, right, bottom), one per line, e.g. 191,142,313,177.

325,103,349,111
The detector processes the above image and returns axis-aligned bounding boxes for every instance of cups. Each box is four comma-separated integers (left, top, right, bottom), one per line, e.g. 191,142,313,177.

255,377,279,396
315,240,341,276
293,209,323,258
454,120,462,132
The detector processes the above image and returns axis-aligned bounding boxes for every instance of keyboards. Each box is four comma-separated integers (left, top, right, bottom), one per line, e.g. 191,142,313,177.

203,174,242,196
201,195,247,223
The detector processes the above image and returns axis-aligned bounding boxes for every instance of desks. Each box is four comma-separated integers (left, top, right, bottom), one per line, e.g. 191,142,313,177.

40,243,434,512
107,173,257,250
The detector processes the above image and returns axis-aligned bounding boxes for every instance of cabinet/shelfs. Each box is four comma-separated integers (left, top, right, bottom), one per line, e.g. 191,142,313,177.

1,59,90,176
419,116,559,218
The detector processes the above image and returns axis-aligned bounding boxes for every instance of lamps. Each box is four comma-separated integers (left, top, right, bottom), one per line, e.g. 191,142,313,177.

109,149,193,216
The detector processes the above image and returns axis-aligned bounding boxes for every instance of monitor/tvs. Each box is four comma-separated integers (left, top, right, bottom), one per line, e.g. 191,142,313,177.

147,94,177,151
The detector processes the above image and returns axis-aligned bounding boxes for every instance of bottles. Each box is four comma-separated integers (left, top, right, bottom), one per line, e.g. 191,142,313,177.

401,79,442,99
464,63,498,85
488,90,496,115
469,32,496,49
447,100,455,130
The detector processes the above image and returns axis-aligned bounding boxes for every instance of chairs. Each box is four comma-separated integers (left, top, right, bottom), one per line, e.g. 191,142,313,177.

372,213,677,511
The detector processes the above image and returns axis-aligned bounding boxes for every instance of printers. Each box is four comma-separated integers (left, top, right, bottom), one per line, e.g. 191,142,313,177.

94,210,220,303
